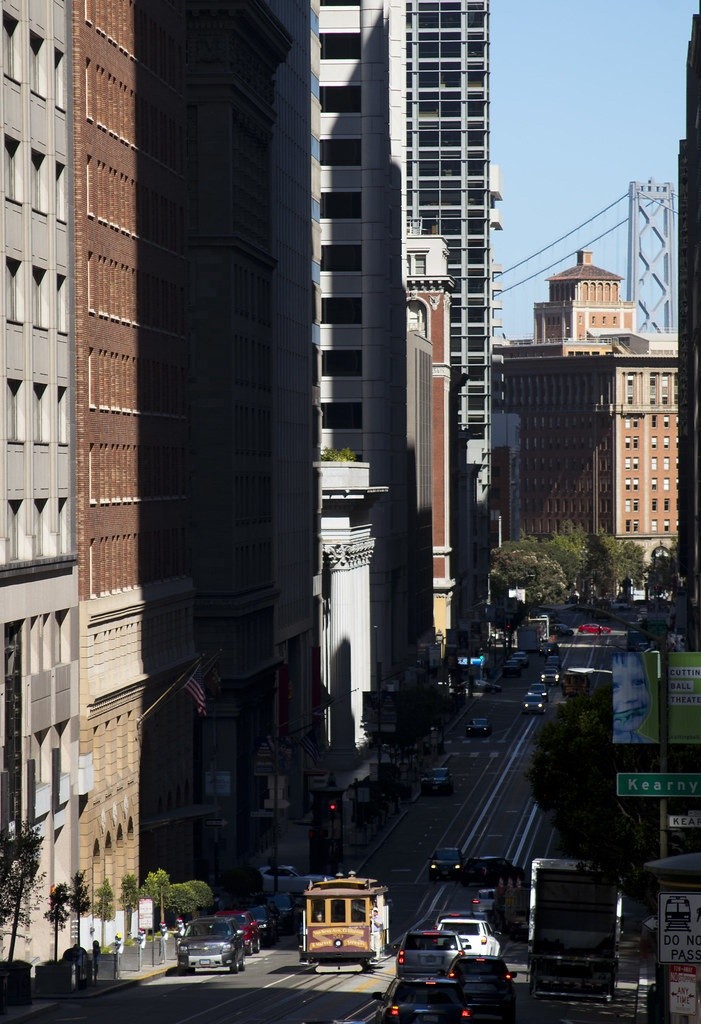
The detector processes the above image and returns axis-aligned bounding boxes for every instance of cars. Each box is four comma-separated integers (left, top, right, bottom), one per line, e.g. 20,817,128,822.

624,605,652,653
499,640,564,715
421,769,454,797
579,623,612,635
461,856,528,888
259,864,337,895
456,679,503,695
548,624,574,637
464,718,492,738
427,847,466,882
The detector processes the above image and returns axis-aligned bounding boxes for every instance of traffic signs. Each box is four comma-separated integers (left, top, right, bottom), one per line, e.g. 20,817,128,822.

640,915,658,932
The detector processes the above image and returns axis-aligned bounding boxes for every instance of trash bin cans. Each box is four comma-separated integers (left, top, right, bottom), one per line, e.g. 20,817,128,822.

0,961,9,1015
6,960,33,1006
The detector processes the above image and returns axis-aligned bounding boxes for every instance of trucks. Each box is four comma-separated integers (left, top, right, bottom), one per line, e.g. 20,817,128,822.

527,863,625,1003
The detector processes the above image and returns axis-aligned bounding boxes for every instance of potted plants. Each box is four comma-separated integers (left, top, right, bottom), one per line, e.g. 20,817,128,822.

35,958,73,994
0,959,34,1005
121,939,140,971
163,932,180,959
87,946,120,980
142,935,164,965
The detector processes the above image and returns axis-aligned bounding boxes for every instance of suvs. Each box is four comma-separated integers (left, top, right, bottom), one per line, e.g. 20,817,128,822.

392,887,531,978
237,903,277,949
262,892,298,935
439,953,518,1024
373,974,475,1024
173,914,245,976
209,909,260,957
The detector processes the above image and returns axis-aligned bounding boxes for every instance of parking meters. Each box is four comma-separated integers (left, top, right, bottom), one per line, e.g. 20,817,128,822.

72,944,81,992
159,922,167,963
135,931,145,970
92,940,100,987
113,932,123,979
175,917,183,933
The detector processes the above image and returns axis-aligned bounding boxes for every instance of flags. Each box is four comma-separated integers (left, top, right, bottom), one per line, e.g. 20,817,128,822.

185,665,207,716
300,730,319,762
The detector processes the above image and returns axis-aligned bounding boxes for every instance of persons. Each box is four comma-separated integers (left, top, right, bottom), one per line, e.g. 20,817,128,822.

269,901,281,941
612,652,651,743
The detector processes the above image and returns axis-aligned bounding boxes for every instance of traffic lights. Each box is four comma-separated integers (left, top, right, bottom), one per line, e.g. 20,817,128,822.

328,799,338,815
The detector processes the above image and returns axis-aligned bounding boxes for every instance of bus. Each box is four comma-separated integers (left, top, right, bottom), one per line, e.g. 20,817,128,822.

298,870,389,974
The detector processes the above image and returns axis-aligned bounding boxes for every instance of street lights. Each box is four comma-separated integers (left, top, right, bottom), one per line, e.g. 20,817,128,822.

539,603,671,1024
